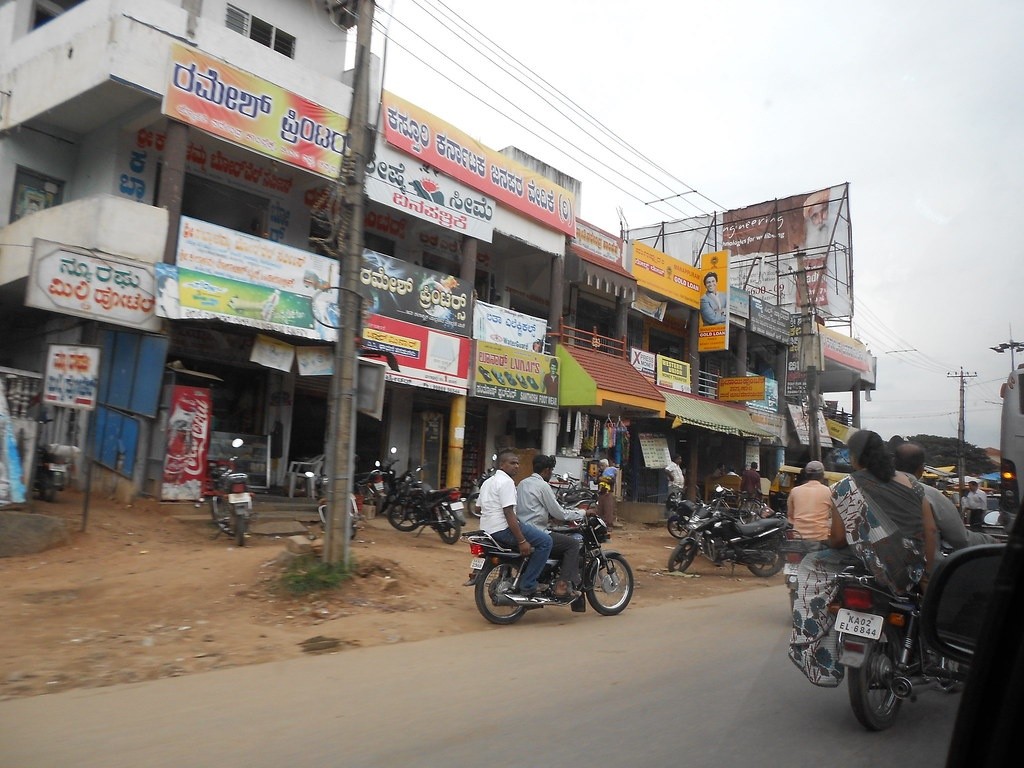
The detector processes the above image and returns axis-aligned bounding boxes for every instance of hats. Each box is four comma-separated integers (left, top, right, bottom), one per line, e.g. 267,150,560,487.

805,460,824,474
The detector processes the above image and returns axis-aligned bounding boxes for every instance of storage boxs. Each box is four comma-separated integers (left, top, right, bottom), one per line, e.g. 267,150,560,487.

362,506,377,519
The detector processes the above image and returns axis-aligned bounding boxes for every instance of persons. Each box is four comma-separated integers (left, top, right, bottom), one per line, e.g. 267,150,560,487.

593,459,617,531
726,467,740,478
769,464,788,512
790,430,1003,687
967,481,987,532
544,358,559,397
700,272,726,326
786,461,833,547
665,454,685,519
803,190,830,254
739,462,760,498
516,454,596,597
712,463,726,480
475,451,553,597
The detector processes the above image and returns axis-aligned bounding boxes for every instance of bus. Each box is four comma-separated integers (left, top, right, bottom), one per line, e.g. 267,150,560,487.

999,369,1024,533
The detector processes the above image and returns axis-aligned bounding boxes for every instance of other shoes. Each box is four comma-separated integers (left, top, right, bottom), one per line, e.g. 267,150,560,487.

608,526,613,532
605,491,610,496
598,490,602,494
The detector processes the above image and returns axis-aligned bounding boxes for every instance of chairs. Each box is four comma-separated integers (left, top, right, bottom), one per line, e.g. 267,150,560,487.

713,475,741,491
285,453,324,499
704,475,716,504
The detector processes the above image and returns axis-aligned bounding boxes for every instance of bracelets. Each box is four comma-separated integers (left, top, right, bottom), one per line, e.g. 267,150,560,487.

519,539,526,544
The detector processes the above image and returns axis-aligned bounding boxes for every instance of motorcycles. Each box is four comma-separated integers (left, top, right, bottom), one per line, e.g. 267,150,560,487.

663,484,792,584
461,471,634,624
782,525,810,617
357,446,606,546
34,416,78,506
305,470,364,541
828,563,966,732
192,439,255,546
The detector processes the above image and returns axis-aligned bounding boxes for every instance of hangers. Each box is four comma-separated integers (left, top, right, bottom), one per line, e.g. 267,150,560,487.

616,416,625,428
605,413,613,424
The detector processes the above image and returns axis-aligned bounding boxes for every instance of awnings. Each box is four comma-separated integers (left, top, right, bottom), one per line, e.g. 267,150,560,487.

660,392,774,442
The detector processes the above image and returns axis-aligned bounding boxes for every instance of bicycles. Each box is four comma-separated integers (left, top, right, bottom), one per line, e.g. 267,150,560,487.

710,482,762,525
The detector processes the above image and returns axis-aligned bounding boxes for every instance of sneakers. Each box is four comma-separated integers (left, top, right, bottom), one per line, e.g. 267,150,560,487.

521,580,549,596
555,580,582,597
